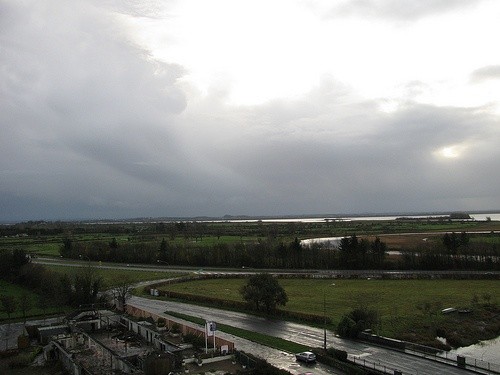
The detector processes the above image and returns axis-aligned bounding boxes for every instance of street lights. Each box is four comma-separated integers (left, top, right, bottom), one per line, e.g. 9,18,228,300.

323,283,336,350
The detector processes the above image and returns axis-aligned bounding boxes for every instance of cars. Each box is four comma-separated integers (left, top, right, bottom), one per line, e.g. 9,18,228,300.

295,352,316,363
